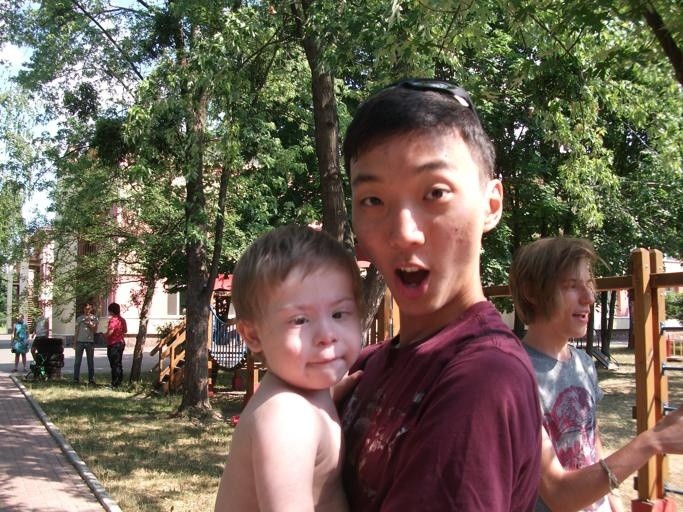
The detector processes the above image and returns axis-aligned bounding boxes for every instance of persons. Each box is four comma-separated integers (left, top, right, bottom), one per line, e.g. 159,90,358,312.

9,313,29,372
71,303,97,385
213,223,364,512
100,302,127,387
507,235,681,511
335,77,542,511
29,307,49,362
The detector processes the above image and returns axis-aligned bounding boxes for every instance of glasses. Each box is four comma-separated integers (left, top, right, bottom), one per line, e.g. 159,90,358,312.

345,80,484,141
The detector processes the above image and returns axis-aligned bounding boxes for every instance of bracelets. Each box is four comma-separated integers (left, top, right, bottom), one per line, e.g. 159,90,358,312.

598,458,620,492
